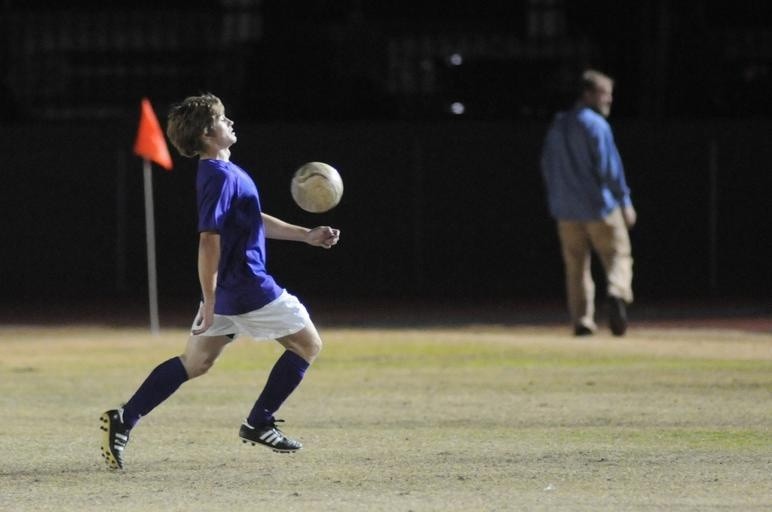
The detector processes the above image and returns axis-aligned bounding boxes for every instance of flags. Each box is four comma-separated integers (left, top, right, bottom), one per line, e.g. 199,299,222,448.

132,98,173,171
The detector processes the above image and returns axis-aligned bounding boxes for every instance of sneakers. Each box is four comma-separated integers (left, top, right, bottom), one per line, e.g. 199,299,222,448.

574,316,597,336
98,406,133,475
604,293,629,337
238,416,304,455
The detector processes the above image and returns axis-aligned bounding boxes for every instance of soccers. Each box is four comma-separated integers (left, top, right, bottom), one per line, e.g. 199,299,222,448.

291,161,343,213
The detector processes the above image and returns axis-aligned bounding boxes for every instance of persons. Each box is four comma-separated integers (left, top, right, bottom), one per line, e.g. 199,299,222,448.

99,93,340,474
540,71,637,336
